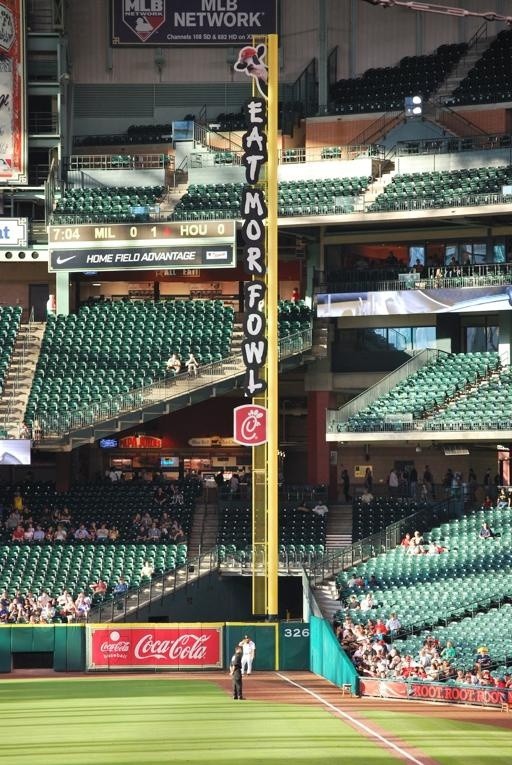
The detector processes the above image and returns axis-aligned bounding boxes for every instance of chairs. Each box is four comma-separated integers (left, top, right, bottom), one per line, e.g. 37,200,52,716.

52,111,246,225
277,263,512,690
277,31,512,217
0,291,250,622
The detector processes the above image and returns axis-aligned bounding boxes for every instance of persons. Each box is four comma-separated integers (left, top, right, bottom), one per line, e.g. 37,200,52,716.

1,464,512,690
289,248,512,304
185,352,199,375
0,576,127,623
229,646,245,701
166,352,182,373
